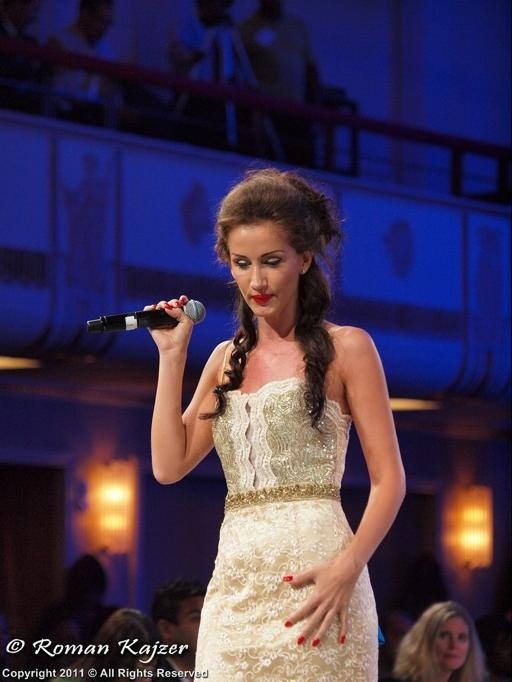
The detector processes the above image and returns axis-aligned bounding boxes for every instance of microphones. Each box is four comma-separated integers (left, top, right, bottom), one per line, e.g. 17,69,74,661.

85,295,209,342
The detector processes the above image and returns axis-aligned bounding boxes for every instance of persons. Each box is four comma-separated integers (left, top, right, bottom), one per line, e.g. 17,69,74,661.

0,545,512,682
137,161,409,682
0,1,351,174
